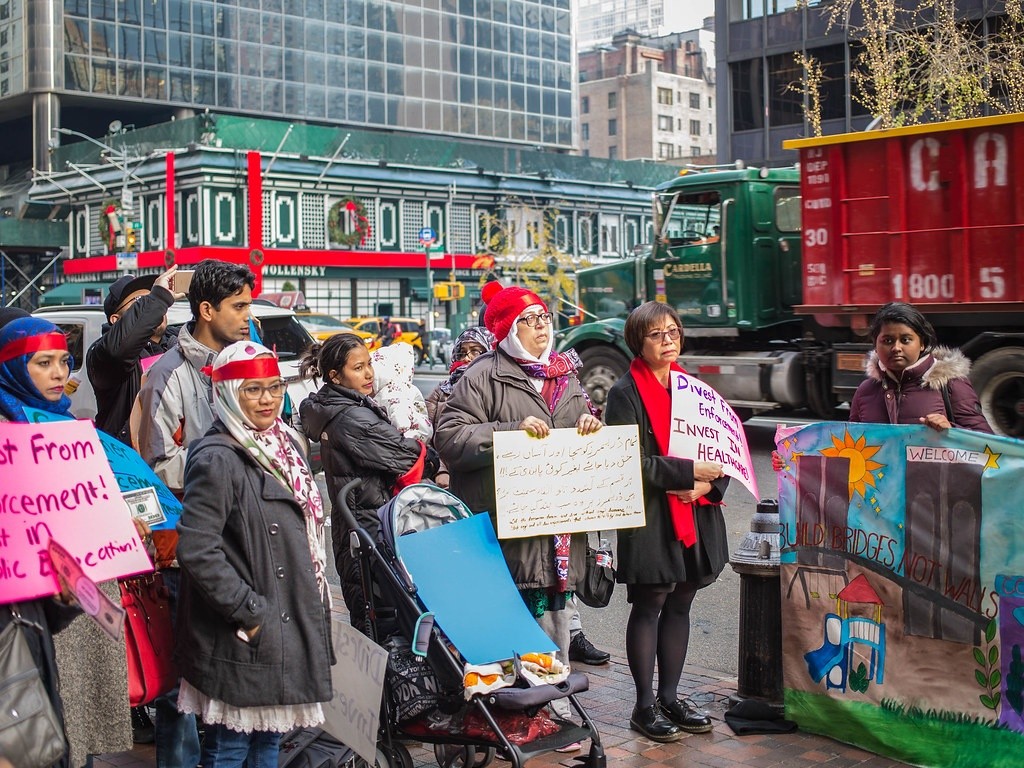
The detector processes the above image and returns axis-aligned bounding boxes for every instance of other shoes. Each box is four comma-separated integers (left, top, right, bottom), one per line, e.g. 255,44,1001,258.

555,741,580,752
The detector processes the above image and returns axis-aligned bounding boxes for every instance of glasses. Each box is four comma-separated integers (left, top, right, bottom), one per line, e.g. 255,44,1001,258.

643,327,682,344
455,350,487,361
112,295,145,314
238,377,288,400
515,311,553,327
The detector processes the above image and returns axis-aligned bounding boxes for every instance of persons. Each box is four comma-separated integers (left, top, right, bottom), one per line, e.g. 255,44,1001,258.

773,300,994,472
0,260,732,768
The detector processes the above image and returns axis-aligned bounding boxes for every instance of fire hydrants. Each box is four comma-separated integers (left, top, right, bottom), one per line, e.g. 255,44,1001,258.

727,499,786,714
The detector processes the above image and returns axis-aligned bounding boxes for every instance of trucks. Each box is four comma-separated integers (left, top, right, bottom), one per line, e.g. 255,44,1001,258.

553,104,1023,439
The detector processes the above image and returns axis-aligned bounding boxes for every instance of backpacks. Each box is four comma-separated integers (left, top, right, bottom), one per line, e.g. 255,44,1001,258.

277,722,381,768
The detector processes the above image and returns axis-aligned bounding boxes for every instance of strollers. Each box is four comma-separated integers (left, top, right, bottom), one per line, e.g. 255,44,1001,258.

337,477,609,768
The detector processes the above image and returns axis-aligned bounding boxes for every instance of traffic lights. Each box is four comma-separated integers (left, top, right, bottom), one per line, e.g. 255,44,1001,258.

434,283,465,300
126,228,136,250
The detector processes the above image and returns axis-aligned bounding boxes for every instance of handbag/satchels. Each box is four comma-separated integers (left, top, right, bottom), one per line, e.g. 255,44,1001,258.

0,619,68,768
113,570,178,707
383,629,448,723
573,544,615,608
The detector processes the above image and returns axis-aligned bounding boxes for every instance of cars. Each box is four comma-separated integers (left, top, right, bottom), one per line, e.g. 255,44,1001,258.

294,311,376,351
347,317,431,367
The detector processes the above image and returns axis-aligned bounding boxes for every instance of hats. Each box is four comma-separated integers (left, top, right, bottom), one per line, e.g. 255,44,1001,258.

104,274,160,318
482,282,548,342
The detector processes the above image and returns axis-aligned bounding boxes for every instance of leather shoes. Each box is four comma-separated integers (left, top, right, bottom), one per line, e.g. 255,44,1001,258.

654,697,714,733
630,701,682,742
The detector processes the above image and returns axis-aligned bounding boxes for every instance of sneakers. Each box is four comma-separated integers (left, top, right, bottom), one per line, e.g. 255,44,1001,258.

568,632,610,664
131,702,155,743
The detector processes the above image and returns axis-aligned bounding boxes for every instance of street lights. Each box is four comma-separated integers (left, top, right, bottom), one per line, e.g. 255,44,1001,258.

51,128,189,275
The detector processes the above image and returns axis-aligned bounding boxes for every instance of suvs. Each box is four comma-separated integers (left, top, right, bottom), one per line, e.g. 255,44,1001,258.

31,296,325,506
